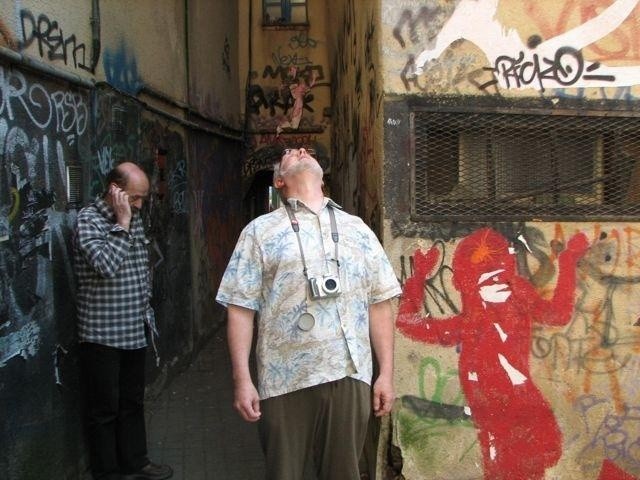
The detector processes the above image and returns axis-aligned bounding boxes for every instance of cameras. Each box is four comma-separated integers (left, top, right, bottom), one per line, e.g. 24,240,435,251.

310,276,341,299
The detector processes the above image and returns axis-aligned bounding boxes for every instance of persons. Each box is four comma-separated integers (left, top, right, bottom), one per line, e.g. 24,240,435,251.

70,161,176,479
215,146,404,479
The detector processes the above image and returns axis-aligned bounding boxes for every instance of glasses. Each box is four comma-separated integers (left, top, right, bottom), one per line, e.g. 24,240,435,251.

282,148,316,156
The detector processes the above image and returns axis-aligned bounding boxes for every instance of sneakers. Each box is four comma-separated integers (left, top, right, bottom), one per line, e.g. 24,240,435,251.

121,461,172,479
